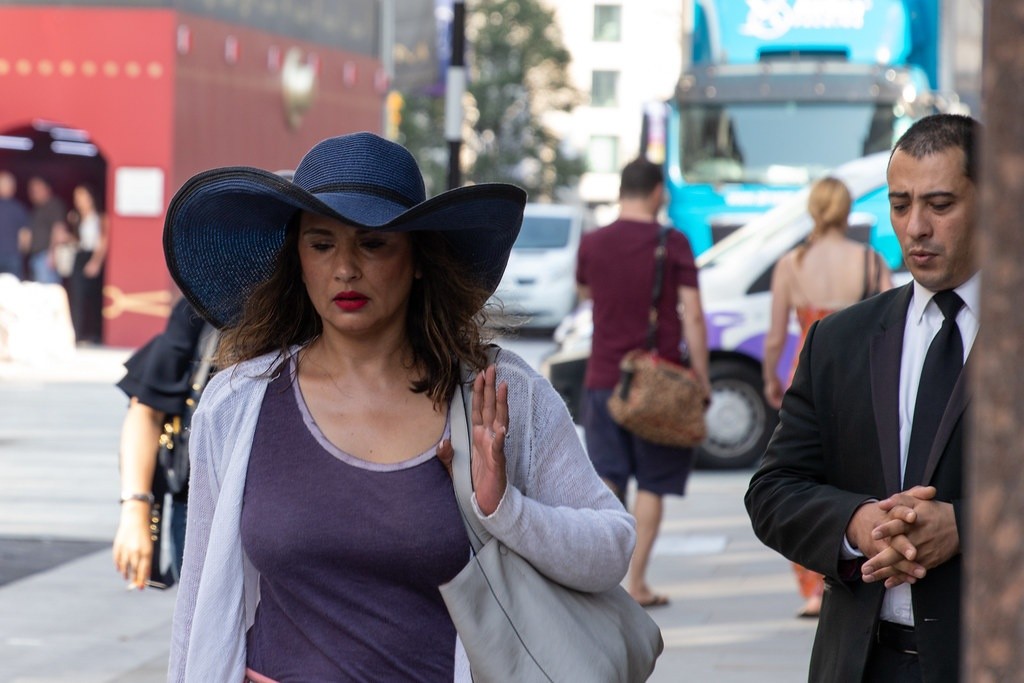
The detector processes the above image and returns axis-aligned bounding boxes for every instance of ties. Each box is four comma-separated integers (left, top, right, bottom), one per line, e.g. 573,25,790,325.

903,292,966,492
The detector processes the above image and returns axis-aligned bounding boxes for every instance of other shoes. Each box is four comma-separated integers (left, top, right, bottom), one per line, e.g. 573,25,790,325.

637,594,670,606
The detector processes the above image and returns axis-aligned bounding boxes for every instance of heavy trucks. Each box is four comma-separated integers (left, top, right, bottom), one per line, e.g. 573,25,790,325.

663,0,939,262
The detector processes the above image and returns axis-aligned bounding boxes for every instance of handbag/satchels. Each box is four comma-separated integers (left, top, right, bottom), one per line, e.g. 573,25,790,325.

133,423,190,588
608,345,708,449
438,537,666,683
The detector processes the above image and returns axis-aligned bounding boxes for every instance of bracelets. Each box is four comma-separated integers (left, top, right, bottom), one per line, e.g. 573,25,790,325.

118,493,155,503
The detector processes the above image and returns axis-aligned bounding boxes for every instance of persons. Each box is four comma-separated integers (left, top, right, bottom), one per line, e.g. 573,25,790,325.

763,178,893,620
0,173,108,343
114,296,242,592
576,158,710,609
745,112,984,683
161,133,636,683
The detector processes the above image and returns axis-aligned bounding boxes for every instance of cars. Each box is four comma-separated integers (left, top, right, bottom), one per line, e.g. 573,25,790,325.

478,205,597,330
541,151,914,472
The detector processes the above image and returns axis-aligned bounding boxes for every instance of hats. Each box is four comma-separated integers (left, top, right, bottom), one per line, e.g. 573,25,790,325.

164,132,526,335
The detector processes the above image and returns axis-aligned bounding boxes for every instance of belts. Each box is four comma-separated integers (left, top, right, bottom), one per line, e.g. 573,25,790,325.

875,624,921,658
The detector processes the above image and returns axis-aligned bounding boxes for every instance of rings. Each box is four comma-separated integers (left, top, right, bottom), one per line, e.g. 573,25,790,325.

492,432,509,439
